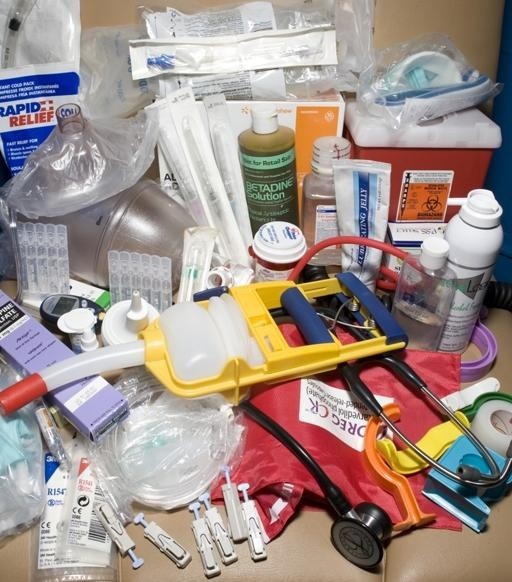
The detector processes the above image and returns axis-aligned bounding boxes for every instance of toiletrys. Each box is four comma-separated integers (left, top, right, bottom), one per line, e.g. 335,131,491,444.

330,157,393,297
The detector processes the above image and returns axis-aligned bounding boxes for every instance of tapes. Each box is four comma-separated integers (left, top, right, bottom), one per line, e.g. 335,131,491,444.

471,399,512,457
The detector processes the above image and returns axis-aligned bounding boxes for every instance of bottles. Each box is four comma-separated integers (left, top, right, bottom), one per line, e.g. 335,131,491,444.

247,221,307,289
432,188,503,356
301,135,351,267
390,236,457,352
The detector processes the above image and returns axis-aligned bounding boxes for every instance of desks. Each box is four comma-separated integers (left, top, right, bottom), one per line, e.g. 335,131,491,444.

1,278,512,582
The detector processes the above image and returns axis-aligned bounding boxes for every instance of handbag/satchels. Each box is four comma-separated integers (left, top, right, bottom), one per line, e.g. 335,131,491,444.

209,324,462,545
